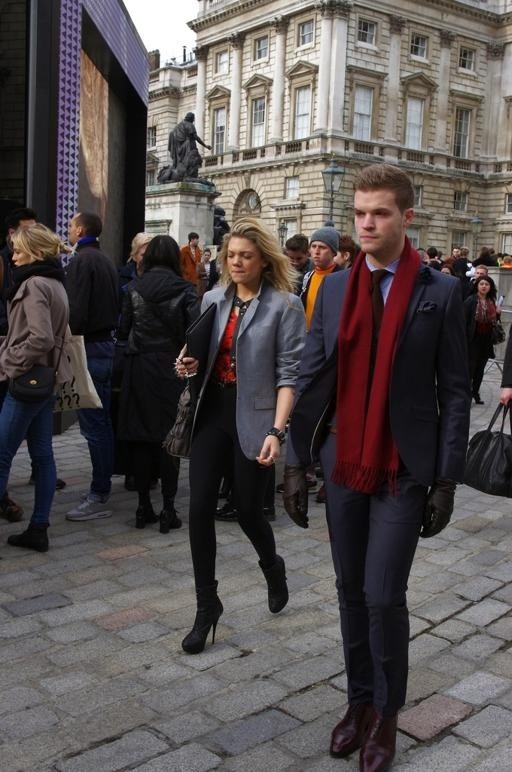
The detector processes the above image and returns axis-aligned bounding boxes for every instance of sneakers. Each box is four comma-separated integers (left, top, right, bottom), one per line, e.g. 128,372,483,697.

316,484,327,503
276,480,320,494
29,469,65,489
262,505,275,521
215,506,238,522
0,490,23,521
8,522,50,552
65,492,112,521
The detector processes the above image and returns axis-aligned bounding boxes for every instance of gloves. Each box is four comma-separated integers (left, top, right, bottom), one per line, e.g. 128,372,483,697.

419,475,457,538
284,464,309,528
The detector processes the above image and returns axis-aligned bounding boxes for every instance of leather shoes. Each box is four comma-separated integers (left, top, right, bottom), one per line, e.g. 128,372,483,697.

360,711,398,772
331,705,372,755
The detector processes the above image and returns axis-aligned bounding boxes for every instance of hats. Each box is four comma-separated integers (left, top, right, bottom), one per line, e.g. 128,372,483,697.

310,220,340,253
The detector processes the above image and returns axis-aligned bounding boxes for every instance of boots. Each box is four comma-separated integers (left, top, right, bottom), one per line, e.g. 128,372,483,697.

258,555,289,613
182,581,223,653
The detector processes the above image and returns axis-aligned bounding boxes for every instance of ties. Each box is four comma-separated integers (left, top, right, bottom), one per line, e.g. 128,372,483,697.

370,270,389,374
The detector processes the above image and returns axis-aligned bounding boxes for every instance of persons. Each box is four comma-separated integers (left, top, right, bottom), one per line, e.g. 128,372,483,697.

1,211,358,656
417,246,511,405
168,113,212,166
283,166,472,771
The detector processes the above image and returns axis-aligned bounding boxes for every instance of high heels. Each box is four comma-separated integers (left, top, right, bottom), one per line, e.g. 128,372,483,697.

135,502,160,529
472,389,484,404
159,508,182,533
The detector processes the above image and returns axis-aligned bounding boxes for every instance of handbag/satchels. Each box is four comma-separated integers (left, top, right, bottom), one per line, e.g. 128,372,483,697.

8,366,56,402
492,318,506,345
53,324,103,413
463,398,512,497
161,379,197,459
